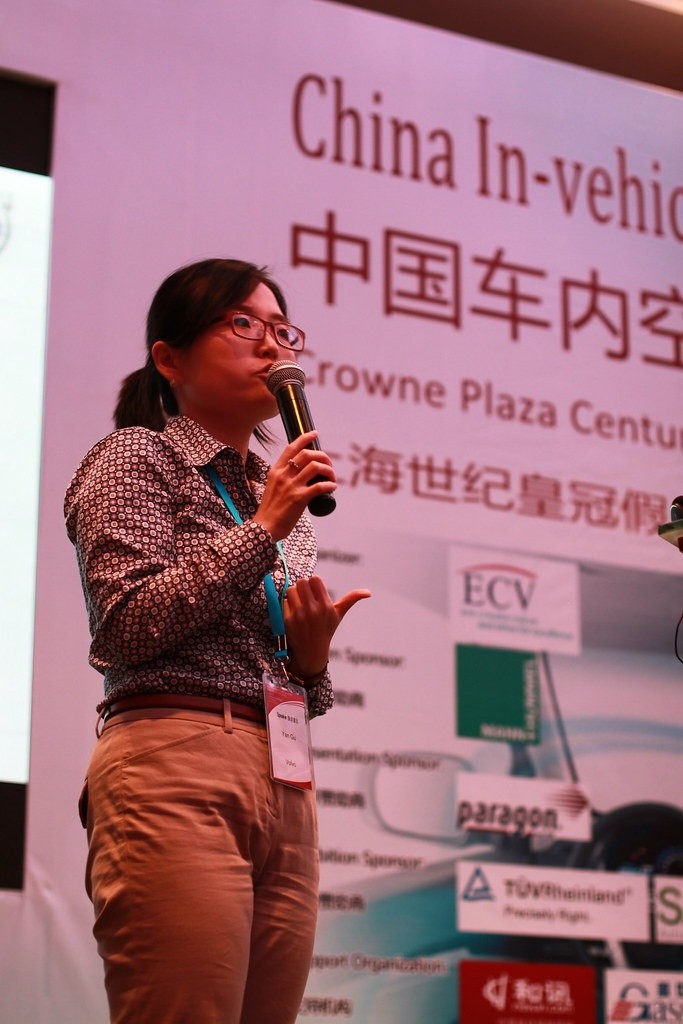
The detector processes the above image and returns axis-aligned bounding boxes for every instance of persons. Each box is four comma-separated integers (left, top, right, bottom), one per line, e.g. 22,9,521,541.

63,260,372,1024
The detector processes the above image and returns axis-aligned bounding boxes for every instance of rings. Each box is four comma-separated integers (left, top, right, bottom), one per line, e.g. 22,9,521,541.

288,459,303,472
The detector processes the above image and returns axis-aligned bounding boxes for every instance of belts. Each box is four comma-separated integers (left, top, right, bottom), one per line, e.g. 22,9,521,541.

102,694,267,722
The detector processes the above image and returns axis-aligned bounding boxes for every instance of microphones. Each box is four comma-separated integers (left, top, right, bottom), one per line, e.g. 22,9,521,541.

264,360,336,517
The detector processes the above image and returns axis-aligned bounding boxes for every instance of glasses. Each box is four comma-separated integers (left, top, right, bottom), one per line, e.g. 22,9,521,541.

219,312,306,351
